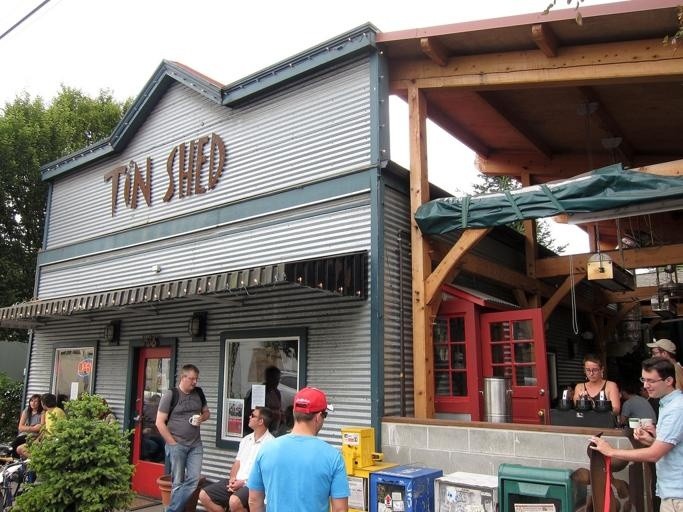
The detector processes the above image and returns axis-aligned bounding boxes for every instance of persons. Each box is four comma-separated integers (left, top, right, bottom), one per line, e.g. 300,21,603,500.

197,406,277,512
155,364,210,512
646,339,683,391
245,363,282,434
617,385,657,425
587,357,683,512
573,354,621,417
245,387,352,512
639,382,659,419
0,392,69,507
97,398,116,426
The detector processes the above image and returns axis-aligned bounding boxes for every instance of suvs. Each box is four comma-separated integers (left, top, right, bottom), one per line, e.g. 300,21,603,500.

278,369,298,428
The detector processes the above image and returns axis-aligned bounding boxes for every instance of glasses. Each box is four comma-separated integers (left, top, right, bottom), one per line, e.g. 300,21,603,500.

640,377,663,383
252,414,257,417
585,368,600,373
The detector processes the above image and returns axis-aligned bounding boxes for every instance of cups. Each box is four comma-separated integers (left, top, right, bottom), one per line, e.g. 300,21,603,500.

189,415,200,426
557,389,612,412
628,417,652,438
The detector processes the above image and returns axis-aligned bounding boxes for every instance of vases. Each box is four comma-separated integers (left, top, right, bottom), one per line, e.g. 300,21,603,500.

156,474,207,512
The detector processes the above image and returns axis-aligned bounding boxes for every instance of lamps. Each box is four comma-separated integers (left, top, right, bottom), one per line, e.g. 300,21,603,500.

650,265,683,318
188,311,208,342
587,217,636,293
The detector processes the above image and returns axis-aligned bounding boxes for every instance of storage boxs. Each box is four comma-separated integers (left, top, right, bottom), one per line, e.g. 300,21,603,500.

103,318,121,346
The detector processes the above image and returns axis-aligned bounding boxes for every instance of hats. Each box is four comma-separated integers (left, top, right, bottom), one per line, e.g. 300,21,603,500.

647,339,676,354
294,387,327,413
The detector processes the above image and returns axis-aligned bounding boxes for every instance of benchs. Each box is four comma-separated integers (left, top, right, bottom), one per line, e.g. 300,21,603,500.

0,441,29,464
